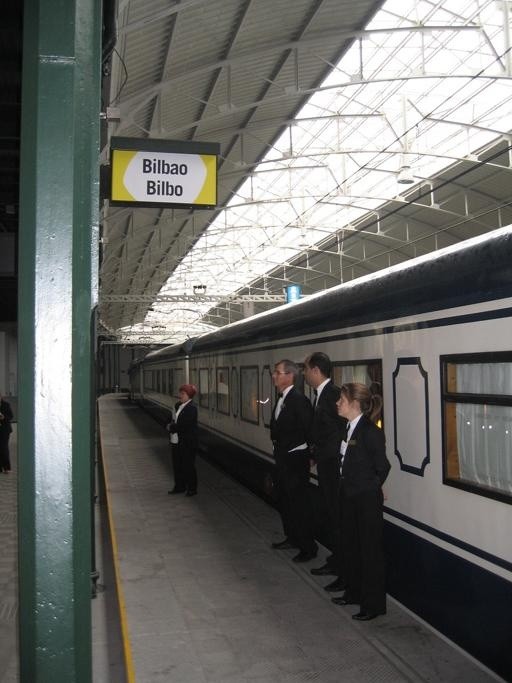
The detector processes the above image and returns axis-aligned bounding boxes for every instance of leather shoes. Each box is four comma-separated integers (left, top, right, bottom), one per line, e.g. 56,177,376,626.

292,551,313,563
168,488,197,497
272,538,297,550
310,563,387,620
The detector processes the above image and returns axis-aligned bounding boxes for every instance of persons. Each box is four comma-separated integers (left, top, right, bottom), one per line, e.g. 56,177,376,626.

330,382,392,621
271,358,318,563
300,351,346,593
166,384,197,495
0,396,11,473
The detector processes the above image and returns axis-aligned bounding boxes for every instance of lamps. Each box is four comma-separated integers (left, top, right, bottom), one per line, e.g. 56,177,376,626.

397,97,415,184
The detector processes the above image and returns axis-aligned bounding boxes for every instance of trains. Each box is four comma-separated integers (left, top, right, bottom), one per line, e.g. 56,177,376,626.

124,220,512,683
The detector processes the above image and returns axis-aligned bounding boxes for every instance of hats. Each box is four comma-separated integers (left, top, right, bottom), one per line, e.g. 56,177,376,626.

179,384,197,397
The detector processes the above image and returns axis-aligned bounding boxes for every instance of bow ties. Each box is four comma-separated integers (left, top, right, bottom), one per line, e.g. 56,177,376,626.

278,391,282,397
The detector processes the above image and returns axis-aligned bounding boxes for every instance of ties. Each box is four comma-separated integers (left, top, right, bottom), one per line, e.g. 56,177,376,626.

344,424,350,443
313,389,318,411
